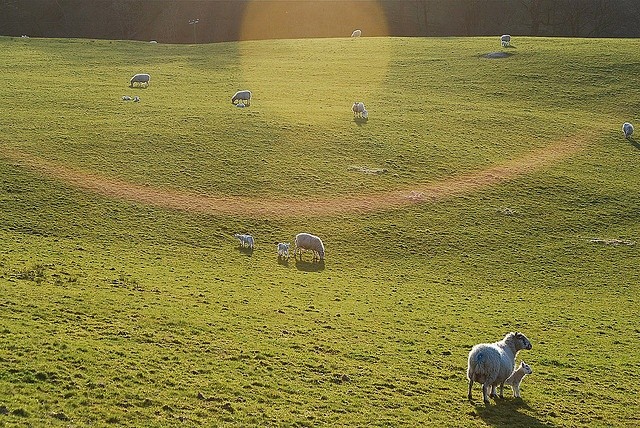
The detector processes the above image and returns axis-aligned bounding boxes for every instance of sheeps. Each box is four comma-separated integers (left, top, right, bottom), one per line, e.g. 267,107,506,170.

501,35,510,42
351,29,361,38
294,233,325,262
232,90,251,106
277,242,290,261
129,74,150,88
466,331,532,403
622,122,634,139
234,233,254,250
362,110,368,118
351,102,364,119
501,41,508,47
481,360,532,398
